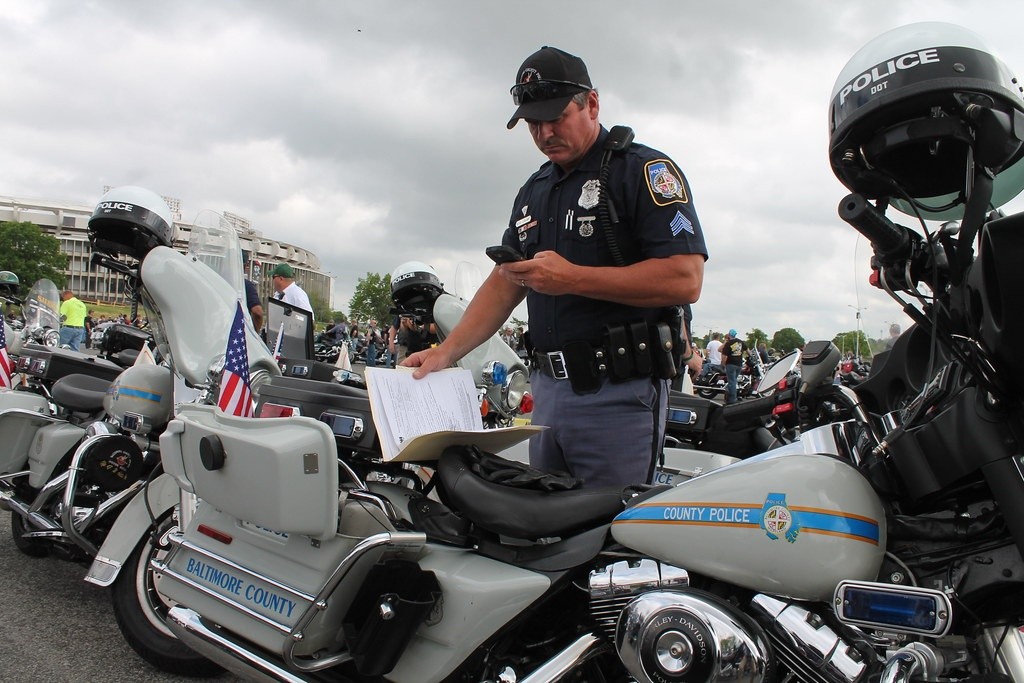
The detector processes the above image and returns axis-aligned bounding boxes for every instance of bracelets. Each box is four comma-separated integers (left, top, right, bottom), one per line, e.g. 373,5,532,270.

683,351,693,361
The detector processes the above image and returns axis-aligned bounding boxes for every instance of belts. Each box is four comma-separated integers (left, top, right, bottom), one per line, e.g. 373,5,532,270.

64,326,83,328
535,351,569,380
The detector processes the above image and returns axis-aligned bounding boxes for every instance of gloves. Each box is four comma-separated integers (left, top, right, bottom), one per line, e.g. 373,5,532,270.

466,443,584,493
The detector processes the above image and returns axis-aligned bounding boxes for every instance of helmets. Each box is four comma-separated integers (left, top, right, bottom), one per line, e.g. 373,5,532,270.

0,270,19,286
104,364,176,435
390,262,443,306
88,186,173,254
692,343,696,347
828,21,1024,221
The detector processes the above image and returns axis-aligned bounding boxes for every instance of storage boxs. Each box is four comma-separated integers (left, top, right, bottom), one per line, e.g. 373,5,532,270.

264,295,361,386
0,386,50,476
158,403,423,657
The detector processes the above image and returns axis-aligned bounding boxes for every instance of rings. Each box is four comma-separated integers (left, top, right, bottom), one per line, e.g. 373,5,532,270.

522,280,524,286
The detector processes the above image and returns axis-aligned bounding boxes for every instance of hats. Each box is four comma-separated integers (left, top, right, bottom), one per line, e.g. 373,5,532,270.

730,329,737,336
88,310,94,314
267,263,294,278
507,46,591,129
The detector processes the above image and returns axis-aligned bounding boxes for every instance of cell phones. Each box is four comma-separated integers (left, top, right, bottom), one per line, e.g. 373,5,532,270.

486,246,526,264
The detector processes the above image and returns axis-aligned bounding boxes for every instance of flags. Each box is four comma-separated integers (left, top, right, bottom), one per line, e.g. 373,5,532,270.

217,303,255,418
271,328,283,364
0,307,12,389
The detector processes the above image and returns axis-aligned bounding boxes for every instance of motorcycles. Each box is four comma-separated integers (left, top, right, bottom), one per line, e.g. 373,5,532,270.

152,21,1024,682
0,275,873,676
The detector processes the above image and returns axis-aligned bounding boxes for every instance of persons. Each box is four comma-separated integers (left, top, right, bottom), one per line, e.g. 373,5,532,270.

262,263,316,329
671,304,702,394
389,314,436,358
85,310,95,350
366,320,377,367
884,325,900,351
383,326,391,367
326,319,358,351
58,291,86,352
99,314,113,323
501,327,528,356
706,329,768,402
121,314,146,328
222,249,263,334
400,44,708,488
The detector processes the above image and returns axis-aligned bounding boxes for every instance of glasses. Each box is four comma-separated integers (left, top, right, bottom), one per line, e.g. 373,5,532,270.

510,79,592,105
273,276,280,279
